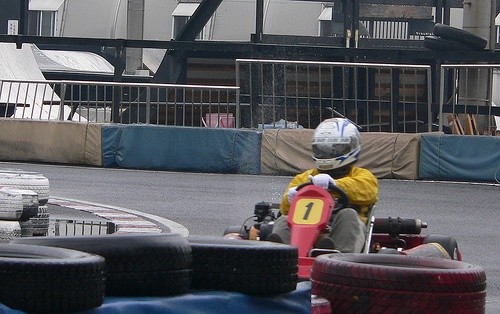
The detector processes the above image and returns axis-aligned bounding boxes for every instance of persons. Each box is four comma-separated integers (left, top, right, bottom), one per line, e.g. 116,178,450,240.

268,117,379,258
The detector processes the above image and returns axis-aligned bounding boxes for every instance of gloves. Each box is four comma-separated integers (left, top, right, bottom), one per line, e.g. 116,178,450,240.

287,187,298,204
307,173,335,190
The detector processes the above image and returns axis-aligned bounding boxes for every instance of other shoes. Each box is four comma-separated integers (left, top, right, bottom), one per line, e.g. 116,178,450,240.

265,233,283,243
318,237,335,255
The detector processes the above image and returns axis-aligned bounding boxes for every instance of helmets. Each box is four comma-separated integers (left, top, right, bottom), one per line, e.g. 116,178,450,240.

311,118,361,170
